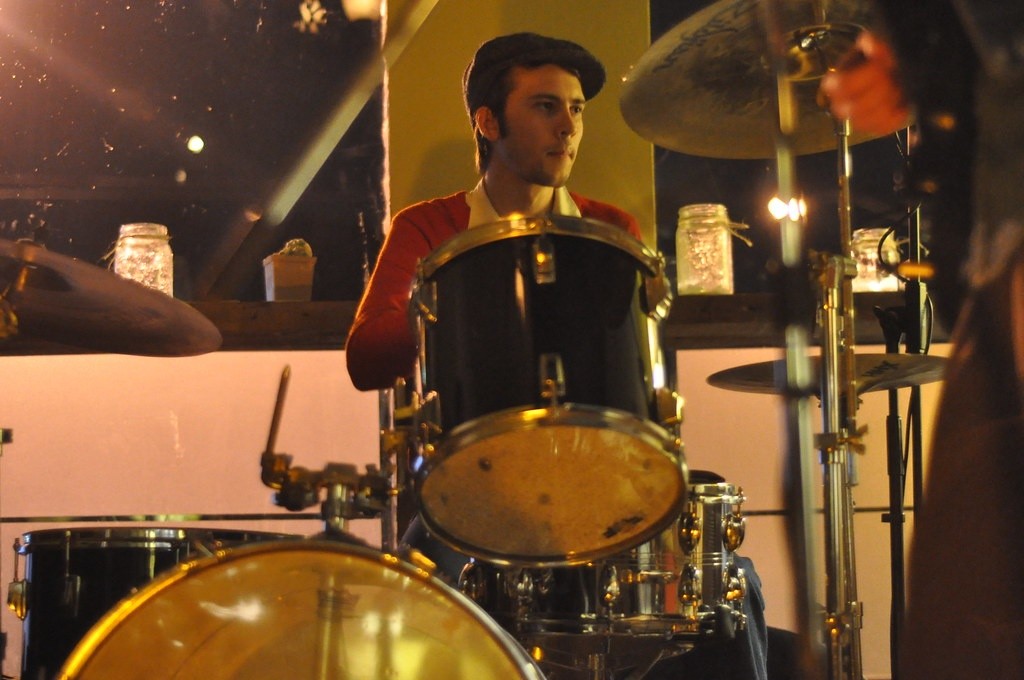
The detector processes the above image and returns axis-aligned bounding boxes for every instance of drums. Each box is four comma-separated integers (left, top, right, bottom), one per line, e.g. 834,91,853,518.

401,213,687,572
56,541,541,680
494,475,749,653
19,514,306,680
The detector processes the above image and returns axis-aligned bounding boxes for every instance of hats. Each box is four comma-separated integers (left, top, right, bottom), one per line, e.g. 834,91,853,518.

462,33,606,105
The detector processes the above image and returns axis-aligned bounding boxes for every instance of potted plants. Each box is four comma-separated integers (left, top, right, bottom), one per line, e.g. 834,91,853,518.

262,238,314,301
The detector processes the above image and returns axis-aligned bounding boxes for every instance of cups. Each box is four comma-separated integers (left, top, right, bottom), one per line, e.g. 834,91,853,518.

114,223,173,299
674,204,735,295
850,228,898,292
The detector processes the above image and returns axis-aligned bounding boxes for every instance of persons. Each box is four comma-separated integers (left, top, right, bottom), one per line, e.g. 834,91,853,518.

346,33,769,680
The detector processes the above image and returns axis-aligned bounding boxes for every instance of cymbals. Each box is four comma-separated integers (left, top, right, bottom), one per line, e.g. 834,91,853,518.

612,0,980,163
2,239,225,359
706,352,953,397
